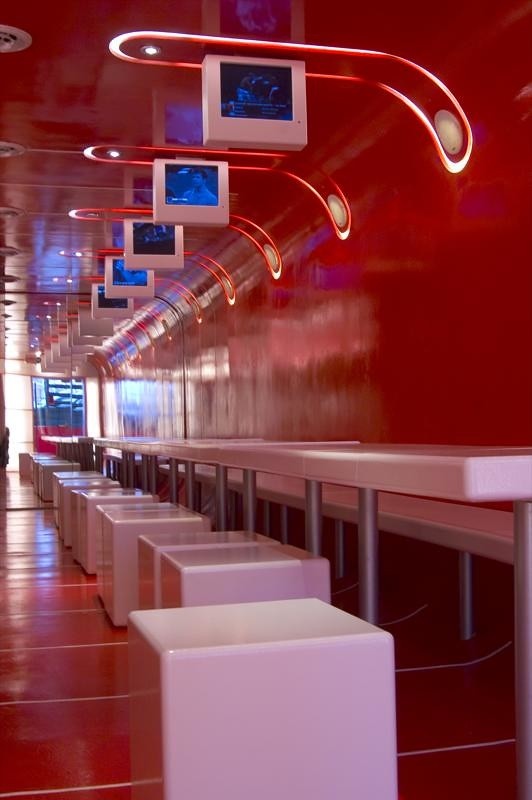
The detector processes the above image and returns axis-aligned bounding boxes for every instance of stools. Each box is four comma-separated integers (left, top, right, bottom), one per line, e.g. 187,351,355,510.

18,452,397,800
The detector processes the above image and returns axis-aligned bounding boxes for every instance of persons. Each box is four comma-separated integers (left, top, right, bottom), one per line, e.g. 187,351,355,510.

240,72,253,88
182,170,217,206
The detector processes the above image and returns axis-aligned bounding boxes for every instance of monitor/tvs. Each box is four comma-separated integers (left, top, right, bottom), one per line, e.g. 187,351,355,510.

198,54,308,151
90,217,184,318
151,158,230,228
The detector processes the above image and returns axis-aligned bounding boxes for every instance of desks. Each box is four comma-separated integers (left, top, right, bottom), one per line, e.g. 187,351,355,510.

41,434,532,800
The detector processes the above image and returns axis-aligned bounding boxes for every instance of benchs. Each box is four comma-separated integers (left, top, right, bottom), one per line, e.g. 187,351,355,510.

105,453,515,643
